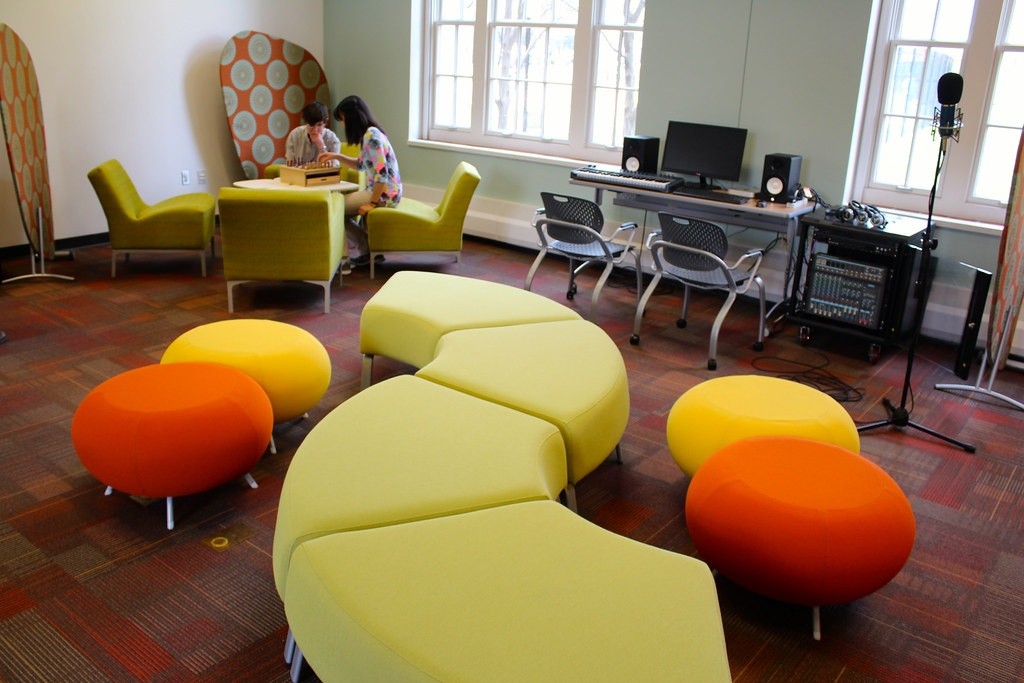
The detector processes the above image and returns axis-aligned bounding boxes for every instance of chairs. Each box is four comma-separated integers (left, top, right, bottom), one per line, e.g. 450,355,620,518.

367,160,482,281
88,159,216,278
524,192,643,323
218,187,345,314
265,141,369,192
629,211,768,371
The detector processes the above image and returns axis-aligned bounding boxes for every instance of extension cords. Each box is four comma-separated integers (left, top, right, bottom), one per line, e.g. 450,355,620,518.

786,197,807,208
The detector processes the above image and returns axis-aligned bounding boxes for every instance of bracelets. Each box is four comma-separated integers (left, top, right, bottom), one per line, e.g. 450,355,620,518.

371,201,378,205
319,145,327,152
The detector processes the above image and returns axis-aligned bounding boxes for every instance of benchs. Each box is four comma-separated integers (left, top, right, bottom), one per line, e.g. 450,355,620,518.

416,318,630,513
272,373,574,665
359,271,583,391
283,499,733,683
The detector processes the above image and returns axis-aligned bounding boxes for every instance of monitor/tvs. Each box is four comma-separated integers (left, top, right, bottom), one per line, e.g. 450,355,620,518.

661,120,748,189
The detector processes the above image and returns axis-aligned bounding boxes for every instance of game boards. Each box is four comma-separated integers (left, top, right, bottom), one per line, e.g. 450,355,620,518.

278,161,342,171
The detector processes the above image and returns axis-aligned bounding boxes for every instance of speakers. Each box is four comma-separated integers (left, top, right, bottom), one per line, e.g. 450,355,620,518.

760,153,802,204
621,135,660,175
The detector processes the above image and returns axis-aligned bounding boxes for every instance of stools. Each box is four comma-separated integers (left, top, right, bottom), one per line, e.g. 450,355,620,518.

667,374,860,509
70,363,274,530
684,435,916,642
161,319,331,453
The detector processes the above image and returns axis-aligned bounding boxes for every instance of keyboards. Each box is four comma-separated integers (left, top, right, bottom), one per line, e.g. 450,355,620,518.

673,187,748,204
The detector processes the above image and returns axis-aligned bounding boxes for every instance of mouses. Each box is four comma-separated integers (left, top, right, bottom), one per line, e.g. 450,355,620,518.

756,200,767,208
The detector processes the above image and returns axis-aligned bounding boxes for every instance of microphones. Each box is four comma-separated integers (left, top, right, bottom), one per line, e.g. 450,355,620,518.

937,73,964,137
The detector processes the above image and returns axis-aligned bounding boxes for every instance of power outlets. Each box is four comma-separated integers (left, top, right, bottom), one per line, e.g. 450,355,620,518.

197,169,207,185
181,171,190,185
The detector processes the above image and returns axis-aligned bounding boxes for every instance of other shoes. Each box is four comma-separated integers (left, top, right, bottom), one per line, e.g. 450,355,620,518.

336,256,351,274
350,254,385,265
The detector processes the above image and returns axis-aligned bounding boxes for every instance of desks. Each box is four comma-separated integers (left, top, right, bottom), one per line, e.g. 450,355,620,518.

568,173,822,338
233,176,361,193
784,205,935,365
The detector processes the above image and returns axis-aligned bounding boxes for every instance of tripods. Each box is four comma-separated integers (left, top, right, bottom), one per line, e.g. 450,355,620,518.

856,137,977,453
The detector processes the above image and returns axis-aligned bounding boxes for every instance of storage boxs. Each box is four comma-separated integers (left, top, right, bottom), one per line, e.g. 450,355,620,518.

278,163,342,186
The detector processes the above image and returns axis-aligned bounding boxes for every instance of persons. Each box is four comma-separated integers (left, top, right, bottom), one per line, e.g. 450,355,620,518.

285,102,340,165
316,96,402,275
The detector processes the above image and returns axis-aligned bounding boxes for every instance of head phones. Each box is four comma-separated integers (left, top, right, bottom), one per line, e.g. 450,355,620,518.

825,200,888,227
